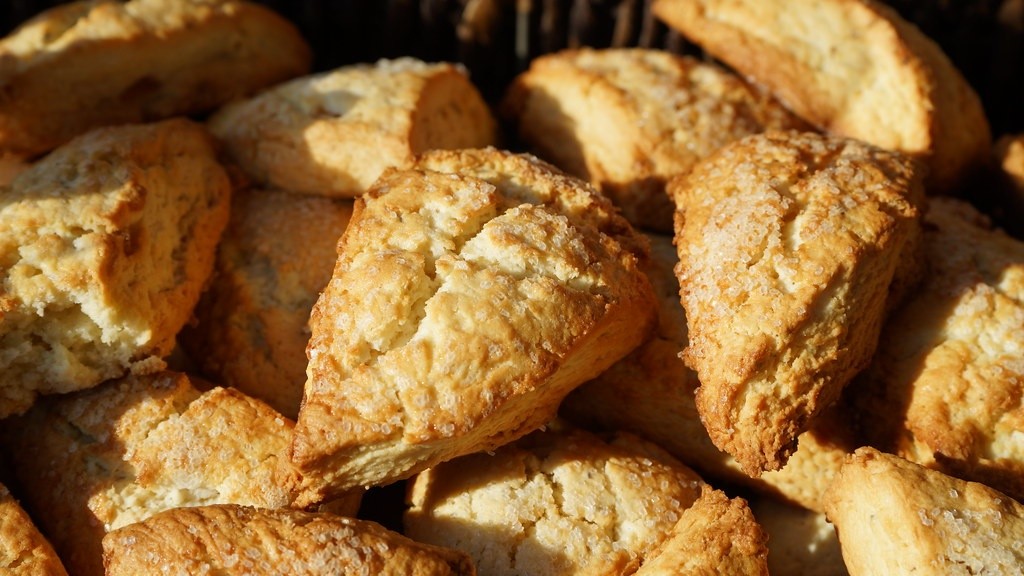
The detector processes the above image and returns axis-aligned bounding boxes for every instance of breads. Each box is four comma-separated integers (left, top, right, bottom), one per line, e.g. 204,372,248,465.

0,0,1024,576
